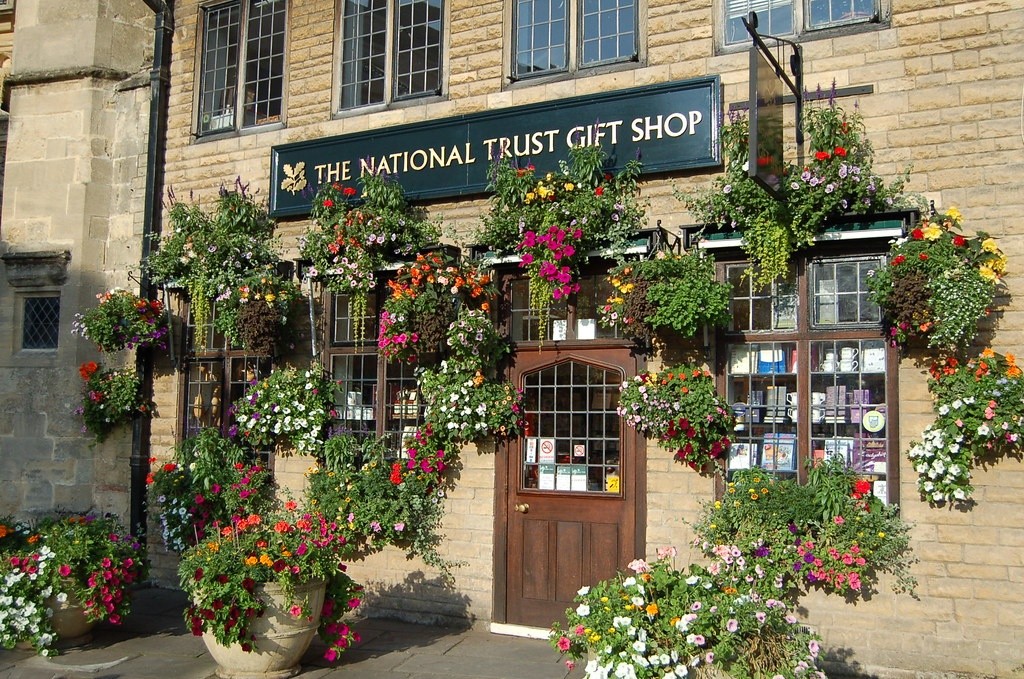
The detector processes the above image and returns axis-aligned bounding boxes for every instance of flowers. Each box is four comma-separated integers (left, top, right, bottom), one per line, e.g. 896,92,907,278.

304,430,470,584
667,77,914,298
864,206,1009,363
141,424,276,557
176,484,370,662
373,249,532,448
0,503,151,662
548,535,829,679
226,359,345,458
681,450,921,601
616,363,737,476
469,116,651,356
65,361,158,452
70,286,171,357
596,243,734,342
125,176,304,363
904,346,1024,505
295,155,443,355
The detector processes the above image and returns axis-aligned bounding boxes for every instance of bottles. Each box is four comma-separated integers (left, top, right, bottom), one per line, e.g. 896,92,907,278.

394,390,418,415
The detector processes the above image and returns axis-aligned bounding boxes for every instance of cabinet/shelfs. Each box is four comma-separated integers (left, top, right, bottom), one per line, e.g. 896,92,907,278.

729,371,887,476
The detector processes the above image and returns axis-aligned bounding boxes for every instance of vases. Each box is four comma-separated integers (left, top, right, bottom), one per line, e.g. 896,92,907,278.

188,576,326,679
15,576,95,654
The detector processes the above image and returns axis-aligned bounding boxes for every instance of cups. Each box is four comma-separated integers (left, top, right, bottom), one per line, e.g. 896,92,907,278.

841,361,858,372
787,391,798,405
787,408,799,423
825,347,838,360
811,392,826,406
841,347,859,360
811,408,826,423
823,361,838,372
347,391,362,410
